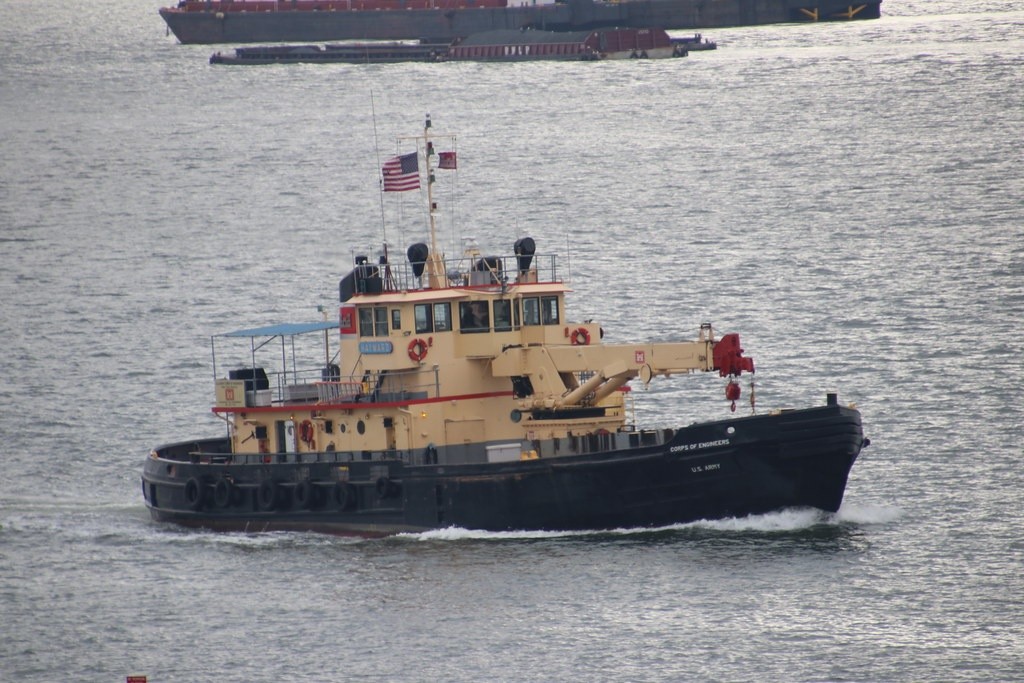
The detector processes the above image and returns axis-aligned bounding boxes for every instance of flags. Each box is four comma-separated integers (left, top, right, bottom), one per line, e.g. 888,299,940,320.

438,152,456,169
381,151,420,192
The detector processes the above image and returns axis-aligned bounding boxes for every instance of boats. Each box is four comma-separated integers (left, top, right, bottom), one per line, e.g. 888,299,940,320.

140,91,872,544
160,0,631,49
210,27,717,68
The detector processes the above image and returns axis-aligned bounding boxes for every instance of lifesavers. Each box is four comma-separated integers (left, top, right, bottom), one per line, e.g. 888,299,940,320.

375,477,391,499
298,420,313,442
256,478,279,512
408,338,428,361
213,479,234,507
332,480,356,511
292,480,313,508
183,477,206,511
570,327,591,345
